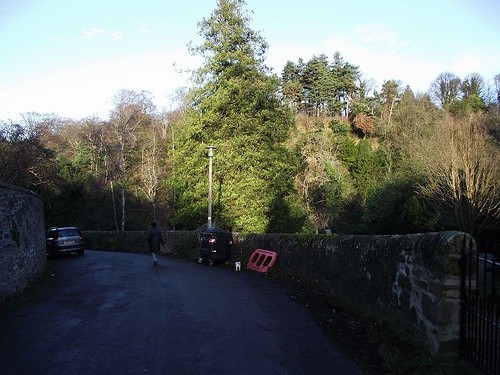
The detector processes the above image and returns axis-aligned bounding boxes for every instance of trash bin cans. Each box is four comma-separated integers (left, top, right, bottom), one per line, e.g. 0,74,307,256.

196,228,233,266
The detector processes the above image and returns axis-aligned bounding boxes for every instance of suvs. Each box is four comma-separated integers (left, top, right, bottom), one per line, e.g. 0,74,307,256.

48,227,84,258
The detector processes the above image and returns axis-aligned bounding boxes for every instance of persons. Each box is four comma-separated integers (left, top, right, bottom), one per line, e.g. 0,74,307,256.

146,221,167,266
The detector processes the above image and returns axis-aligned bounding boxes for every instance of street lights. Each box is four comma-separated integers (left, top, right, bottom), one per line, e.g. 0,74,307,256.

205,146,217,228
